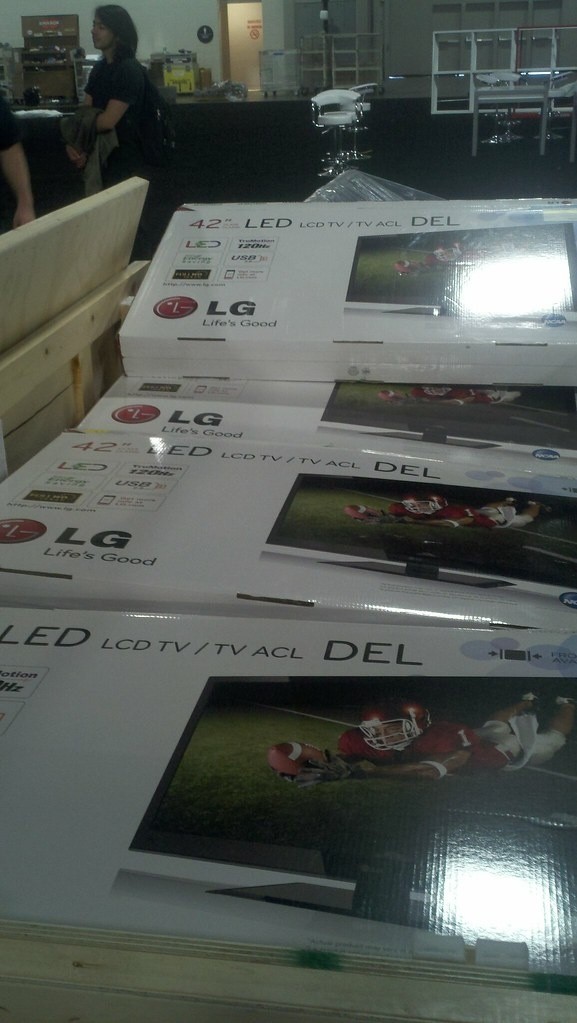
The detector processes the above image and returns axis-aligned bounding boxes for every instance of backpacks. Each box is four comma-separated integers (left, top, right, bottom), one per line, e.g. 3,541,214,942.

112,54,171,141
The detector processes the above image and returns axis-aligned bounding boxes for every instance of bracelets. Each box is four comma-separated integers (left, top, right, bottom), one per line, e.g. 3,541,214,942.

420,760,447,777
455,399,463,406
445,519,459,527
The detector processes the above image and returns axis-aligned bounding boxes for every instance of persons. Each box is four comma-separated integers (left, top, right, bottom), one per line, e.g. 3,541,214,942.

404,244,464,272
384,384,521,406
292,686,577,788
0,97,35,228
355,488,552,529
65,6,152,260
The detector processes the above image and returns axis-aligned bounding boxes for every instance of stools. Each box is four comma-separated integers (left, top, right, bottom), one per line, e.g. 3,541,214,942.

311,81,379,177
476,70,567,143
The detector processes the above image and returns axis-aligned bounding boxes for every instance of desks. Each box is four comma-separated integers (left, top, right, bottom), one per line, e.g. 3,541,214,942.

471,82,577,161
11,96,327,180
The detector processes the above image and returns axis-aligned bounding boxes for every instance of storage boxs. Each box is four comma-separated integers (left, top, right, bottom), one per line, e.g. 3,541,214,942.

0,195,577,1023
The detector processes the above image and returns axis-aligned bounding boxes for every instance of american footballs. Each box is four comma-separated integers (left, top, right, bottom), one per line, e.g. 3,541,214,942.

343,504,383,521
394,261,413,272
267,741,328,777
377,390,405,402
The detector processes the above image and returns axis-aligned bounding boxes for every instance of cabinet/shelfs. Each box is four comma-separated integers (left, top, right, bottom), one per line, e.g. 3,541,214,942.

430,27,577,113
259,32,383,97
21,13,81,99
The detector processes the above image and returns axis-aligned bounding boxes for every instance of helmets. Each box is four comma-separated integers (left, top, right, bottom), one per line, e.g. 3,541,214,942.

360,699,430,754
414,384,452,398
434,242,463,262
399,490,446,517
